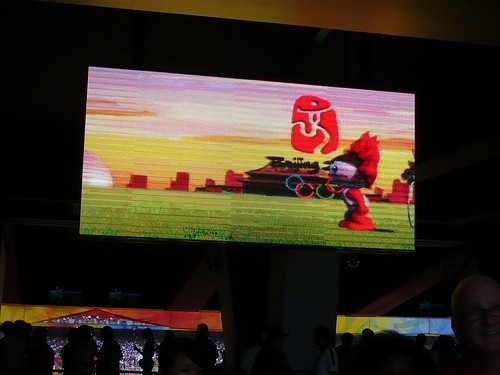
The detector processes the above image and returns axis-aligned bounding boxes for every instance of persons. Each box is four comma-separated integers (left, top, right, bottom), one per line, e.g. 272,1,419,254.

0,321,463,375
451,274,500,375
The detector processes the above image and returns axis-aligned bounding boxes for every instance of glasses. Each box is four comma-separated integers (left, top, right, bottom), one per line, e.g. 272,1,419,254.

458,304,500,320
